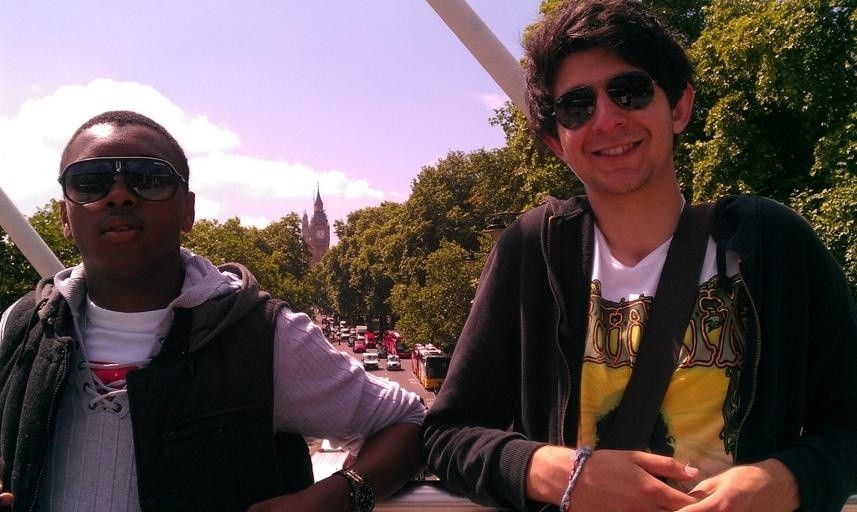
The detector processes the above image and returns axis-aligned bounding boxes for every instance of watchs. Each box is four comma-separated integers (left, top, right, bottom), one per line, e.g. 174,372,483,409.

334,465,376,512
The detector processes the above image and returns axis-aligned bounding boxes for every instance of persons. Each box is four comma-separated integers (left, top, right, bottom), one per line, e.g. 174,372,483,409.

419,1,855,511
0,110,432,512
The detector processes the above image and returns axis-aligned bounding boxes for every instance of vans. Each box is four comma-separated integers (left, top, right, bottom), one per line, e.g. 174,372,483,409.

360,352,381,370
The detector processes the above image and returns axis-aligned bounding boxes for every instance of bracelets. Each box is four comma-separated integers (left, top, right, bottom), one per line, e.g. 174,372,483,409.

560,445,592,509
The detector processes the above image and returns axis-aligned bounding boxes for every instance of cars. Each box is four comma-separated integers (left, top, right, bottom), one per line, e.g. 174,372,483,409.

386,355,402,371
324,318,387,358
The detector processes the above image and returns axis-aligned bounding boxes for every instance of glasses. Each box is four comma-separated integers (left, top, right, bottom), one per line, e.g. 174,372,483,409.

58,156,188,203
551,71,654,129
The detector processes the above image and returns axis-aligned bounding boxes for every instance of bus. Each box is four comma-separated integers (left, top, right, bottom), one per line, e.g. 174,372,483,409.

382,328,413,358
411,342,451,390
